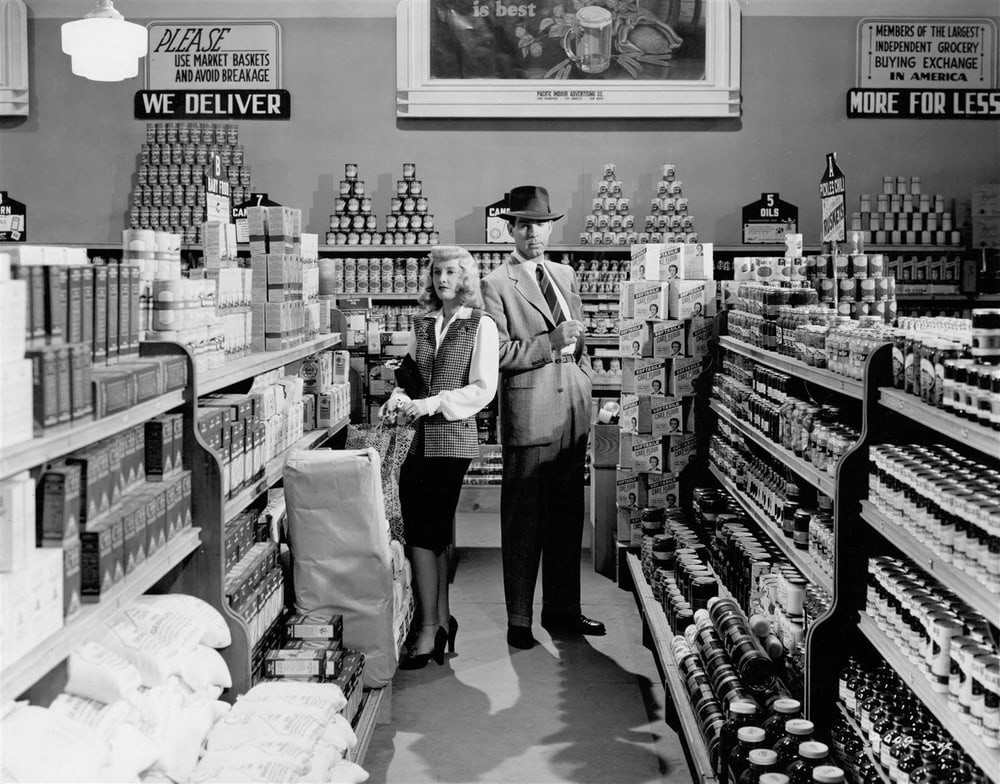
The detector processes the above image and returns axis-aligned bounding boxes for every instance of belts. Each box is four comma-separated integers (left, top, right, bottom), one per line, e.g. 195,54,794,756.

562,355,577,362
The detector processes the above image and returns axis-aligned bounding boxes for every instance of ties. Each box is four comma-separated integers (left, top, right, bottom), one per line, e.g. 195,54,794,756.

537,264,567,328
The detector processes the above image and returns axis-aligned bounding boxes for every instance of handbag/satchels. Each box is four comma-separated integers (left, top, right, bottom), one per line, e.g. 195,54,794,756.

345,413,416,544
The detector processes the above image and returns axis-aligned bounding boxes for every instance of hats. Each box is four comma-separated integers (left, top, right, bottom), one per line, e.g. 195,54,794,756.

495,185,564,222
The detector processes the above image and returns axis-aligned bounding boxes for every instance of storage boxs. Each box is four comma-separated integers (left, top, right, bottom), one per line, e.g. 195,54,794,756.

11,208,716,625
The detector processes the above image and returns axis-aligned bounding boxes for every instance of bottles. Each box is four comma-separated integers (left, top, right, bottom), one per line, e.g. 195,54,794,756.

478,406,498,445
641,310,999,784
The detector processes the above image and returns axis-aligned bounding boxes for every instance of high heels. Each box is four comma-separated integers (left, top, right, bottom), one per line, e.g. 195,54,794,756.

400,625,447,669
445,616,458,652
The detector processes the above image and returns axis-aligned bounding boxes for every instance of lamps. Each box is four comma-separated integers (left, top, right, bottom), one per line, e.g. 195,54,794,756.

61,0,148,81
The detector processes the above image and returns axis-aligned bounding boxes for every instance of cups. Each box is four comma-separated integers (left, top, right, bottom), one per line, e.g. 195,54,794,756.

732,230,896,324
563,6,612,74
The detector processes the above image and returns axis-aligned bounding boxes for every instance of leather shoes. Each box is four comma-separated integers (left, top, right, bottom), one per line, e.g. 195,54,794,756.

506,621,533,650
565,615,605,635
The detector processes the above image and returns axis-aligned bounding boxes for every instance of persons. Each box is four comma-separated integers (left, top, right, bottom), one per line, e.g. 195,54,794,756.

482,185,607,648
376,244,501,670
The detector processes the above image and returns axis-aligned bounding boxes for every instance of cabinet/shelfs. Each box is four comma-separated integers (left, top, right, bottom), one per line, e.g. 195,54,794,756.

0,243,1000,784
